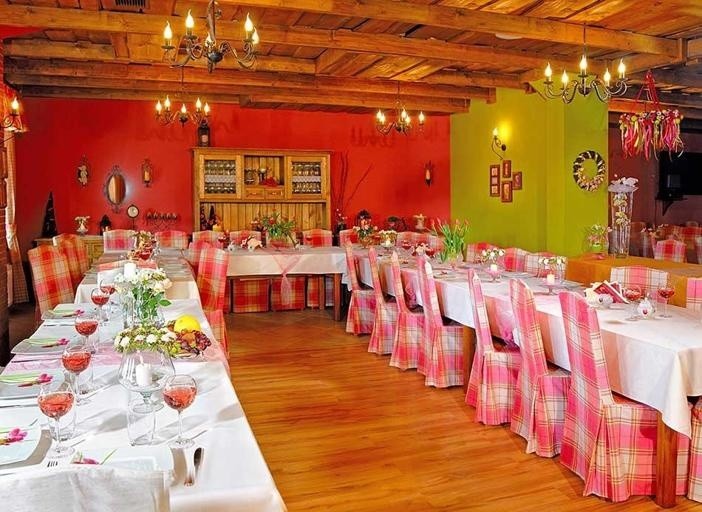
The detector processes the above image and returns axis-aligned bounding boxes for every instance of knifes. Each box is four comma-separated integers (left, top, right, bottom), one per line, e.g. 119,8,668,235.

192,446,202,483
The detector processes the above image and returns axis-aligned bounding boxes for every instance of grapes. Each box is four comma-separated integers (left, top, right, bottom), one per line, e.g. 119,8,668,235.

177,330,210,355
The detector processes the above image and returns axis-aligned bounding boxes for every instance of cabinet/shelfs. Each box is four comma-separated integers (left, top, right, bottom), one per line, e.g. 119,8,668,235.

284,149,331,202
244,185,285,202
192,147,244,202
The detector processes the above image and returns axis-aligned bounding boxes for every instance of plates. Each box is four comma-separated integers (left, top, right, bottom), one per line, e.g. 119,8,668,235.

596,311,628,323
158,247,192,277
72,439,174,489
0,309,80,399
82,249,128,275
127,205,139,218
396,260,580,288
1,423,42,466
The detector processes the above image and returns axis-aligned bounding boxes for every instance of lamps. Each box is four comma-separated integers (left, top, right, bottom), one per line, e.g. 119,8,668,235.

0,96,22,128
375,80,426,135
424,163,432,185
543,22,629,104
141,160,152,185
160,0,260,74
154,61,214,128
490,127,505,160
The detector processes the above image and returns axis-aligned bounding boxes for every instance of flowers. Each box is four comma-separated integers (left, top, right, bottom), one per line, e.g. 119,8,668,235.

573,151,605,190
613,172,632,228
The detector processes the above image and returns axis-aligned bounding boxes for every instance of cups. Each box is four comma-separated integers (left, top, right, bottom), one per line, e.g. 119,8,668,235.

291,164,321,193
205,161,236,194
128,399,157,447
645,290,656,313
140,230,160,267
244,167,256,184
257,167,269,184
48,406,75,440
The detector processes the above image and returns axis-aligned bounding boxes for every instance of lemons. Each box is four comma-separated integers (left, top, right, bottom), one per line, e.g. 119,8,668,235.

174,315,201,332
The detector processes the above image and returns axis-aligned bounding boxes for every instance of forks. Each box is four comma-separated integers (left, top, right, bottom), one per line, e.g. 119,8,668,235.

45,460,60,468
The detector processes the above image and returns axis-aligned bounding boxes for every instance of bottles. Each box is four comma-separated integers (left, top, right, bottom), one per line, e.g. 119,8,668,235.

198,120,210,147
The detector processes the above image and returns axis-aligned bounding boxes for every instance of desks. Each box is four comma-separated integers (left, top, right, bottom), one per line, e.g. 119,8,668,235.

0,299,287,512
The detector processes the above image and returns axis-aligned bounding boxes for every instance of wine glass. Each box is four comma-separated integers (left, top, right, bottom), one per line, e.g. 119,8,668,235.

62,277,117,407
117,345,176,413
657,281,676,318
374,234,434,266
40,381,74,457
621,283,641,322
162,372,197,449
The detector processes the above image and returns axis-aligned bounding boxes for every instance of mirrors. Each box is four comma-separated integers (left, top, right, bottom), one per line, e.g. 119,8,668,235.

103,165,130,213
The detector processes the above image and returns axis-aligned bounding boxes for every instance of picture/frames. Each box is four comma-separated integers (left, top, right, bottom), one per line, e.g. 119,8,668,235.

490,160,522,202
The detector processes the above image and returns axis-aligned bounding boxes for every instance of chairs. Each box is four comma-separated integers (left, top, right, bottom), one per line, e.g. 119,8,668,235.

309,222,702,512
25,230,232,362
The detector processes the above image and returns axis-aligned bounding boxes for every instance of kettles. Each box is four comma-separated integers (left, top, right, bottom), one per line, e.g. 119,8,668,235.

99,216,111,236
